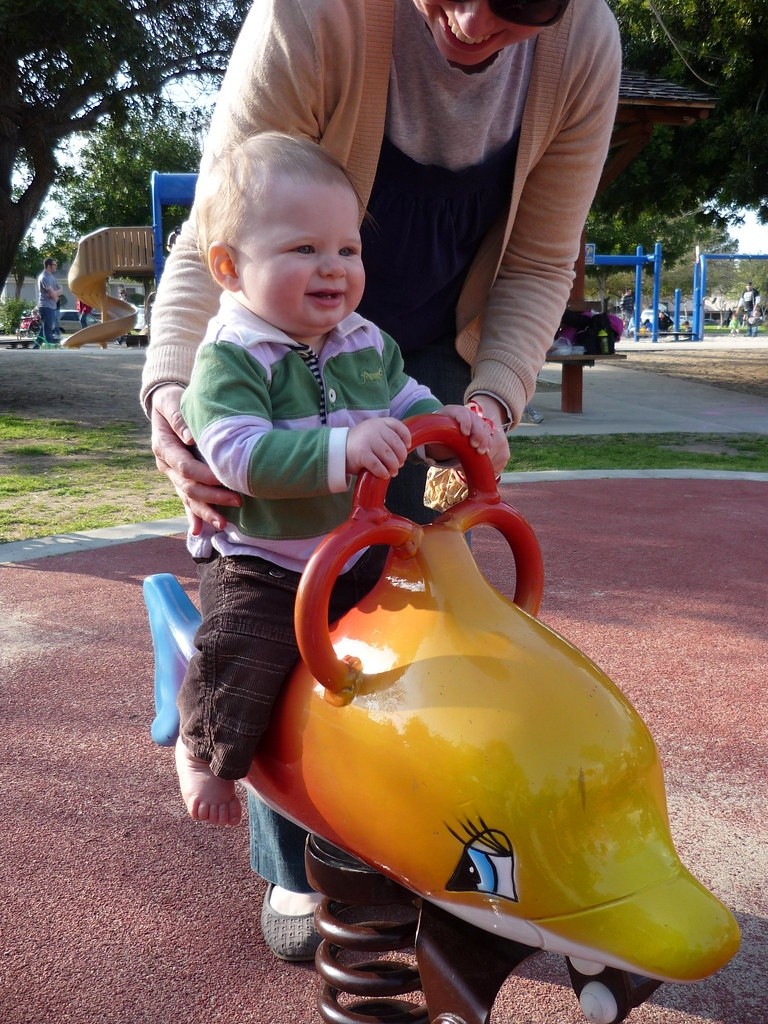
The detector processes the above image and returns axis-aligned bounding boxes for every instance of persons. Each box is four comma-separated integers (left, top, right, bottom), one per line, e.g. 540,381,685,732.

523,404,544,424
619,282,761,339
174,129,498,826
113,289,127,345
75,298,93,329
138,0,626,962
30,258,64,349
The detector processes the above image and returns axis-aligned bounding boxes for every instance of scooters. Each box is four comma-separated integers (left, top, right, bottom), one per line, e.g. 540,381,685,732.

15,310,43,338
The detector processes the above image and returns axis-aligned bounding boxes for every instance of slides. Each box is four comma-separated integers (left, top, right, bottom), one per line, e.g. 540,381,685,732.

58,256,139,349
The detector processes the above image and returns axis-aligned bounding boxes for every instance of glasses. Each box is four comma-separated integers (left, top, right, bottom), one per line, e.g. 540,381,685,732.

449,0,570,27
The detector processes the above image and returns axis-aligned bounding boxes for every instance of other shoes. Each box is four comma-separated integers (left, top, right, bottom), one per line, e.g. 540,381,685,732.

261,883,324,961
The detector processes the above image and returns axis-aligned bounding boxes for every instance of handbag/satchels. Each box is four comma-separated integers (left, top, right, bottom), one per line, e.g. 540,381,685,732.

546,337,585,356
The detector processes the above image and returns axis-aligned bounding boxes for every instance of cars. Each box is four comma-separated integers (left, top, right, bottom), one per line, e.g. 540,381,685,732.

680,316,693,326
131,305,145,330
58,304,103,335
639,309,654,325
